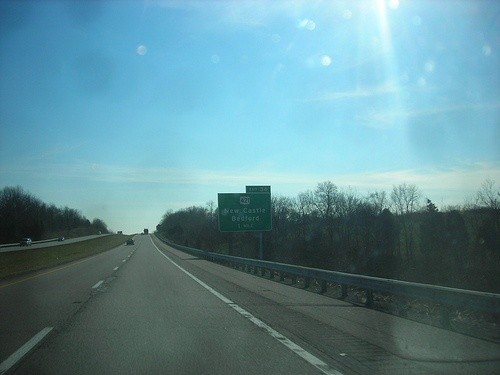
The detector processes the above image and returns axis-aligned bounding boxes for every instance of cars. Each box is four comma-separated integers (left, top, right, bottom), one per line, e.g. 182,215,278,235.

57,228,149,241
127,238,135,245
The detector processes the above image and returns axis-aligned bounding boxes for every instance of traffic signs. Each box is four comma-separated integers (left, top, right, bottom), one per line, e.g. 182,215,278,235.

218,192,272,232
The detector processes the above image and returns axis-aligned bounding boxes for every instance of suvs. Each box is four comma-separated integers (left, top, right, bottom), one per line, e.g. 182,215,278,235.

20,238,32,246
